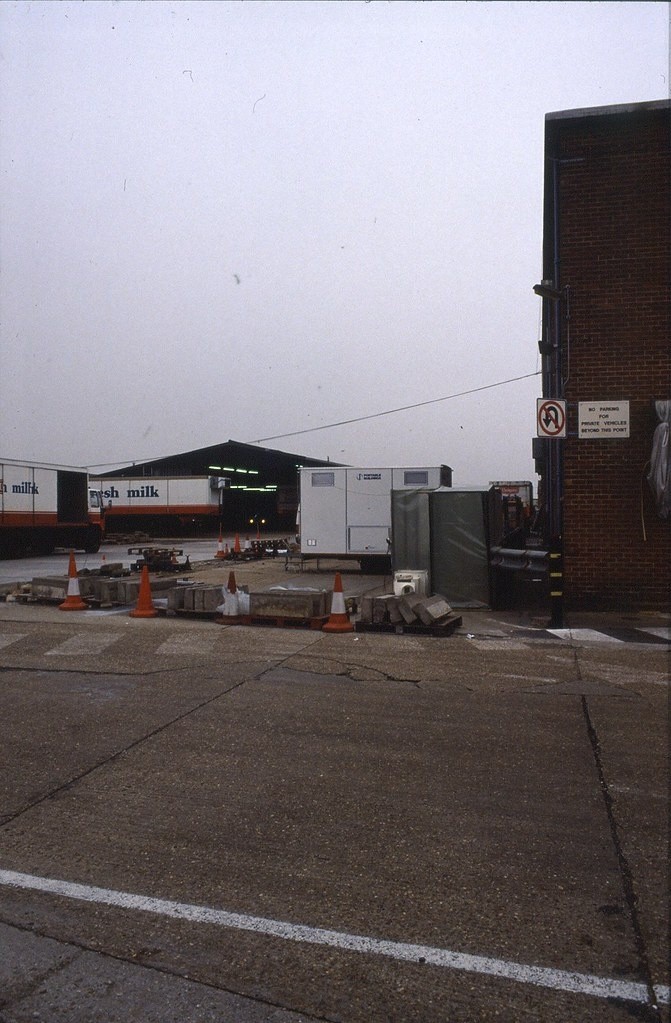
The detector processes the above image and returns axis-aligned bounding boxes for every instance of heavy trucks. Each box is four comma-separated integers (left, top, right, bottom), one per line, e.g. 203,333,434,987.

0,457,113,559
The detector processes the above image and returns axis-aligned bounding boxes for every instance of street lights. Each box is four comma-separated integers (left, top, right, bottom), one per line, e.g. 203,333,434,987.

170,547,179,563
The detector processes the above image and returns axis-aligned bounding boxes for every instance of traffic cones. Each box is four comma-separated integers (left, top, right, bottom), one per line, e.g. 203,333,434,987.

225,543,229,556
215,570,244,626
213,535,226,558
59,559,90,611
128,565,158,618
102,554,106,566
254,530,261,553
322,570,353,633
234,533,241,552
62,551,79,576
